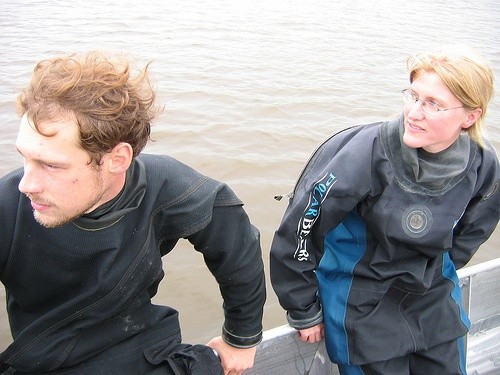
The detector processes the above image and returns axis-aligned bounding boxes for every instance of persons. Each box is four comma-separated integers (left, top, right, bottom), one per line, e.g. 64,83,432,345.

0,51,267,375
269,54,500,375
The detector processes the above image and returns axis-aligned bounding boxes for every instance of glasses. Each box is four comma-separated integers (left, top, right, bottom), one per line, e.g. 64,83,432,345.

402,88,467,114
